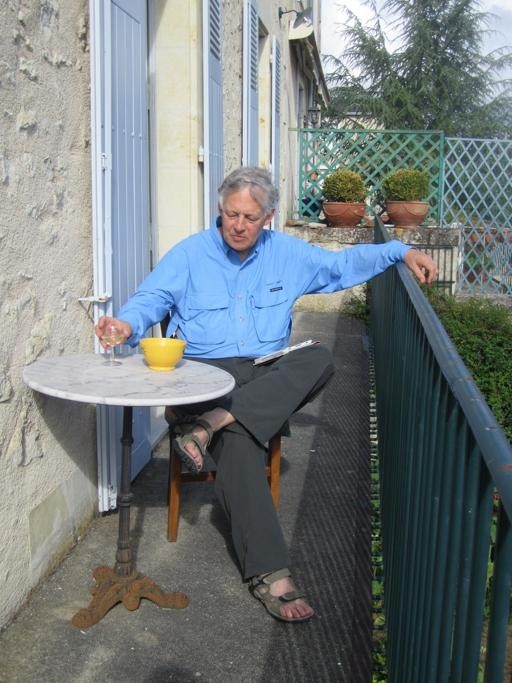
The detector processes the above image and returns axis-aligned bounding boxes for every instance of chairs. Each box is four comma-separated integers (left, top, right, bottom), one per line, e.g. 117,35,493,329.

161,315,281,542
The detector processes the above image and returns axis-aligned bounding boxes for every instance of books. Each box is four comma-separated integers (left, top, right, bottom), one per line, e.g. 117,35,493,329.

253,339,319,365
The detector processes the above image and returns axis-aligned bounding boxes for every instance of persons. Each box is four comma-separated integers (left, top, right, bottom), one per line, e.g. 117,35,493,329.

95,166,439,622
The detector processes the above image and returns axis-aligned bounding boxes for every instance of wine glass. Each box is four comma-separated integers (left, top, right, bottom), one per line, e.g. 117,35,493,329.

100,320,126,367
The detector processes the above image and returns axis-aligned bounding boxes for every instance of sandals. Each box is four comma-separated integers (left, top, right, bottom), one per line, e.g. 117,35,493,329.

248,568,314,622
169,420,213,475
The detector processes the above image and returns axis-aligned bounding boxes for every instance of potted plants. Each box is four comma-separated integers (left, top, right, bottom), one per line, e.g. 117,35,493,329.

382,167,430,228
321,168,365,227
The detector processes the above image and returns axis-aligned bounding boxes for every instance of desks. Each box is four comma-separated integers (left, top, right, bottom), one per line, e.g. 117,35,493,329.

22,349,235,626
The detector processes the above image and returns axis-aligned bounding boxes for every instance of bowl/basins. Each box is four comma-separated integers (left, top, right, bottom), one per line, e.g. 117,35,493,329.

138,337,187,371
